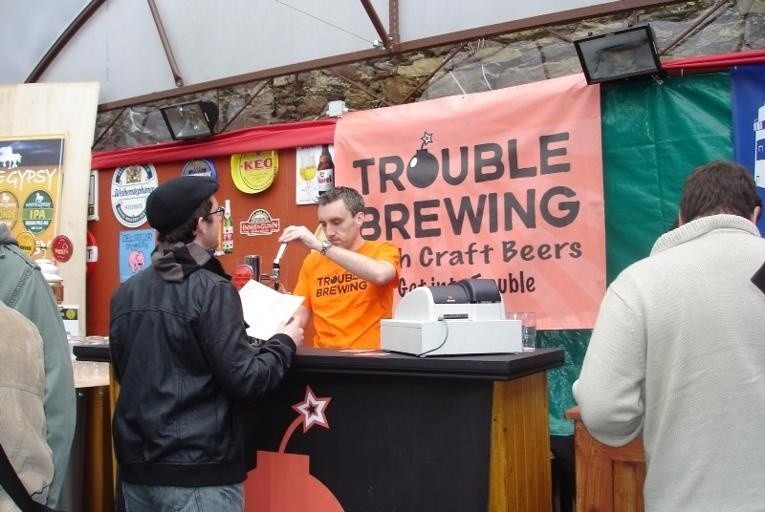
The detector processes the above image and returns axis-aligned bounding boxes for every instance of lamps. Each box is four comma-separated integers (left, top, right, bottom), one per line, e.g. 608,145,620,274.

158,98,219,141
572,22,663,89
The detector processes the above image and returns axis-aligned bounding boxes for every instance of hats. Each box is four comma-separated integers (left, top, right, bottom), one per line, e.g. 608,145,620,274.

146,176,218,229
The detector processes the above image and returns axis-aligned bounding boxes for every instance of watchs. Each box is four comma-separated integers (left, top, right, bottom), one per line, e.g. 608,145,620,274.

319,239,332,257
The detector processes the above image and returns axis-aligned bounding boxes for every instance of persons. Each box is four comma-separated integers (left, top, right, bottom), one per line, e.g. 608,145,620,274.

0,222,79,512
106,173,305,512
277,186,402,352
572,159,764,512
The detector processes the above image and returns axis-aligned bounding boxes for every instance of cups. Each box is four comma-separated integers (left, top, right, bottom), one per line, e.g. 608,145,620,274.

505,311,537,352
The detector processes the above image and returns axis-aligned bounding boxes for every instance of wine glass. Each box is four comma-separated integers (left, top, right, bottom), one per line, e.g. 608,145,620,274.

299,151,318,196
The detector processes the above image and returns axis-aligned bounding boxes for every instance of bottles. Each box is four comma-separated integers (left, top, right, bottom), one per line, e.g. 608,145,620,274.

222,198,236,255
319,143,336,197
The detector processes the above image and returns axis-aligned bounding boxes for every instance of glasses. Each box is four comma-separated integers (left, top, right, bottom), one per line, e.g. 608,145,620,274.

204,206,225,216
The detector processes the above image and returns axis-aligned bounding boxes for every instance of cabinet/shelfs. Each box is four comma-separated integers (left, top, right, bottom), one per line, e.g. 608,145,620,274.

71,341,567,511
561,404,646,511
